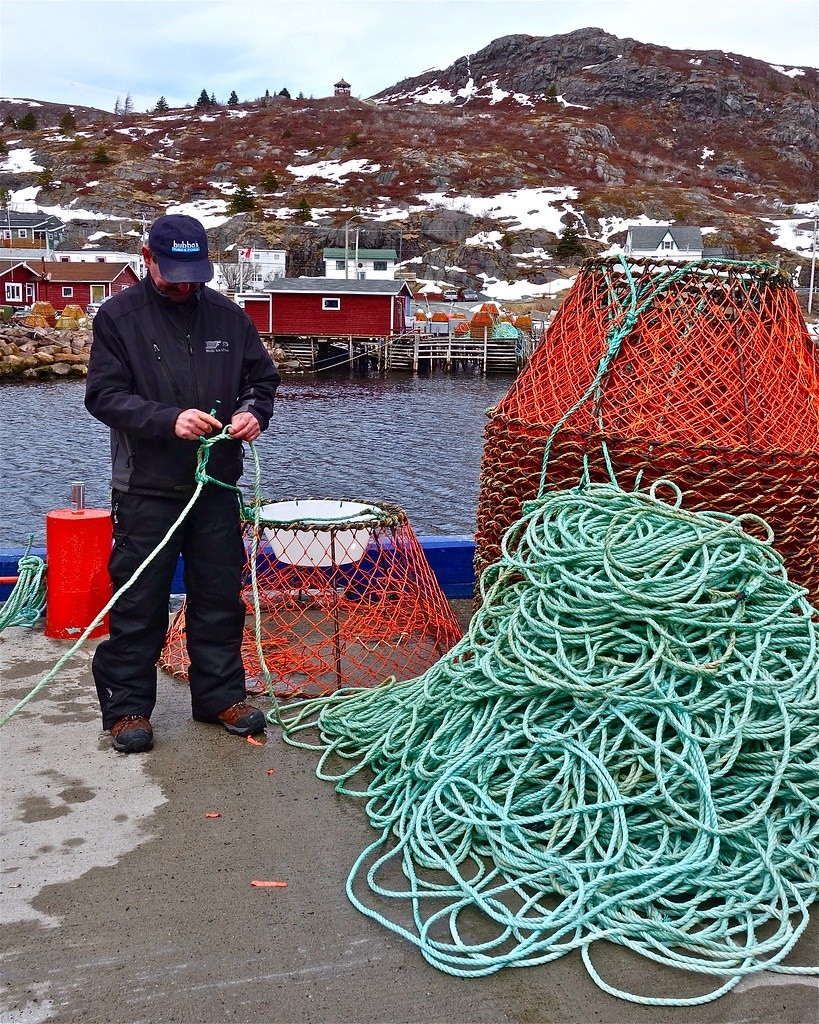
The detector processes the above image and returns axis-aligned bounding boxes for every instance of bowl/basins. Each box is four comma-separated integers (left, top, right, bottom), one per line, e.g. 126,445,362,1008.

259,501,381,567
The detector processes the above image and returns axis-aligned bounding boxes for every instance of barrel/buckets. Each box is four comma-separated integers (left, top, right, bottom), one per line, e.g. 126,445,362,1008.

47,508,112,637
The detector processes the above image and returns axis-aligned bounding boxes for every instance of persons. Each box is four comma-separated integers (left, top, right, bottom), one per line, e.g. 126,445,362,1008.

83,214,281,754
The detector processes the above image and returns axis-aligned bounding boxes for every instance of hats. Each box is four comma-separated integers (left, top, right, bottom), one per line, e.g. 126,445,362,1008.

148,213,213,283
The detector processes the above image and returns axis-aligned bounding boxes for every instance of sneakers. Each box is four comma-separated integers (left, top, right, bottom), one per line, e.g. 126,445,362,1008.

112,716,153,752
217,700,267,736
